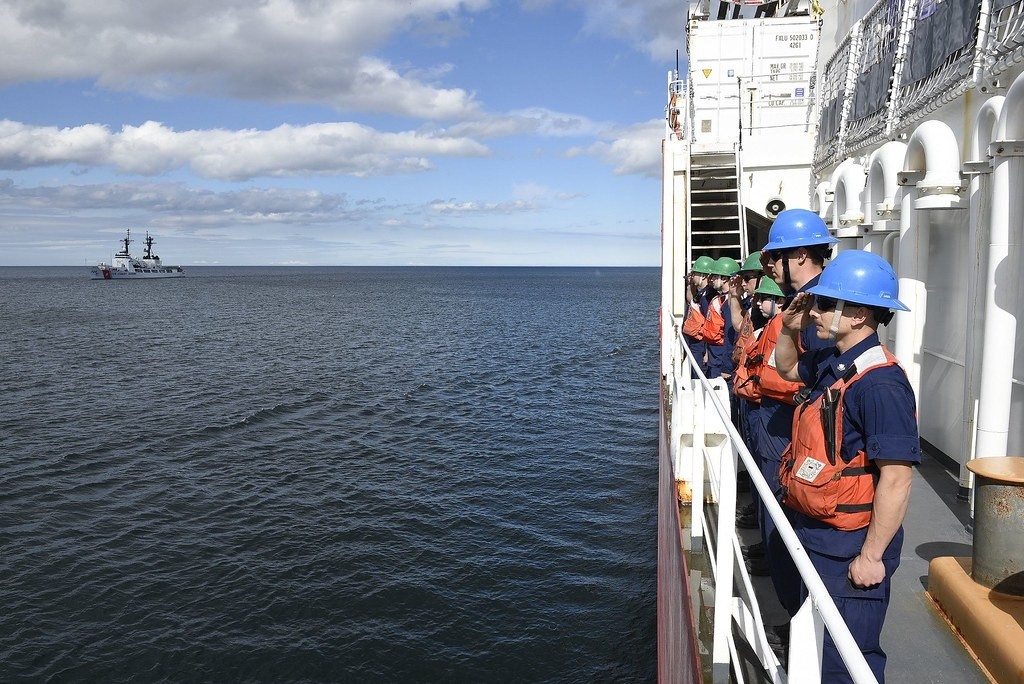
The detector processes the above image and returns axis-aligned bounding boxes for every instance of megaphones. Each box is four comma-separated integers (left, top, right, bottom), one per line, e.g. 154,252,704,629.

765,198,786,219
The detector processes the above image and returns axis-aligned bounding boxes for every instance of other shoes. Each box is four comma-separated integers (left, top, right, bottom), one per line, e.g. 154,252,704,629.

764,622,789,668
736,503,757,517
737,471,751,493
735,513,759,529
745,558,770,576
740,542,767,560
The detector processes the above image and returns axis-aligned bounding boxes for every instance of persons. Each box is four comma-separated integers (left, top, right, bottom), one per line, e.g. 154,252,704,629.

682,208,921,684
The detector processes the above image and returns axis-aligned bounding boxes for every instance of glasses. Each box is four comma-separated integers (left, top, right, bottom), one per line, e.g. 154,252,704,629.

768,249,796,261
743,275,759,282
816,298,868,311
760,296,772,302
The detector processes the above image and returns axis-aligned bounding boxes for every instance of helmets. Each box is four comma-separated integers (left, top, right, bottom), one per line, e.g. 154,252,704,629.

740,251,764,270
762,209,842,250
755,275,786,297
806,250,911,311
691,256,715,274
711,257,740,276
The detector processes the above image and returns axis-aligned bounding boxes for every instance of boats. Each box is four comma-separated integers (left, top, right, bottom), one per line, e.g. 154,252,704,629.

86,228,186,280
